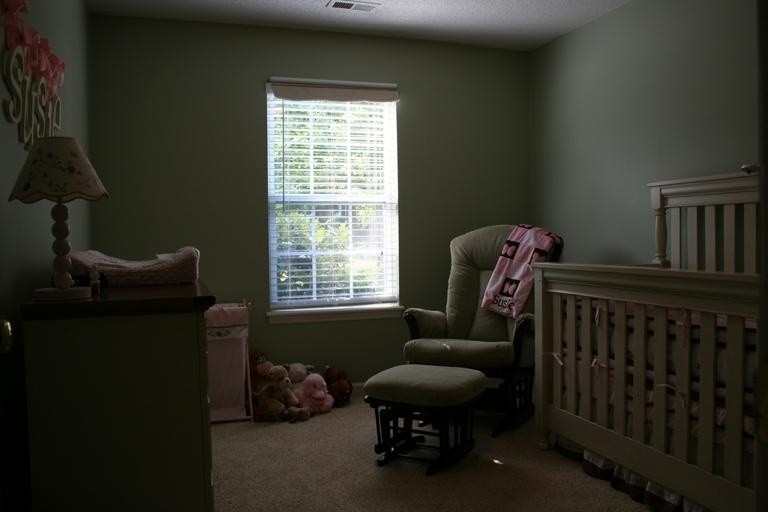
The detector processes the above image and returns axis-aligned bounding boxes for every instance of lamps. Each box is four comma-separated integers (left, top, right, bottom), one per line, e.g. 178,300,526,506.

6,133,110,301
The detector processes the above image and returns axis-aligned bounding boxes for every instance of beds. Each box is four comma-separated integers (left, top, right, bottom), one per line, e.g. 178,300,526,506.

530,172,766,511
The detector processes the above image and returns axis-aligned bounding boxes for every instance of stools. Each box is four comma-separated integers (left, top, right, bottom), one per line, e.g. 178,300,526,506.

362,362,486,480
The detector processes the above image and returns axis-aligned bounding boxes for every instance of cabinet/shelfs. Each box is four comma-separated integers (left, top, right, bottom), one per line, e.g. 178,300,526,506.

21,280,218,512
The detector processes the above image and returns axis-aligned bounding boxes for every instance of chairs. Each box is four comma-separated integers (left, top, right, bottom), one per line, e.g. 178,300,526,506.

398,223,563,432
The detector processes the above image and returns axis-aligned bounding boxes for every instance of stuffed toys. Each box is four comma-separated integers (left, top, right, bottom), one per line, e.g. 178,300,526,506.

245,353,353,423
